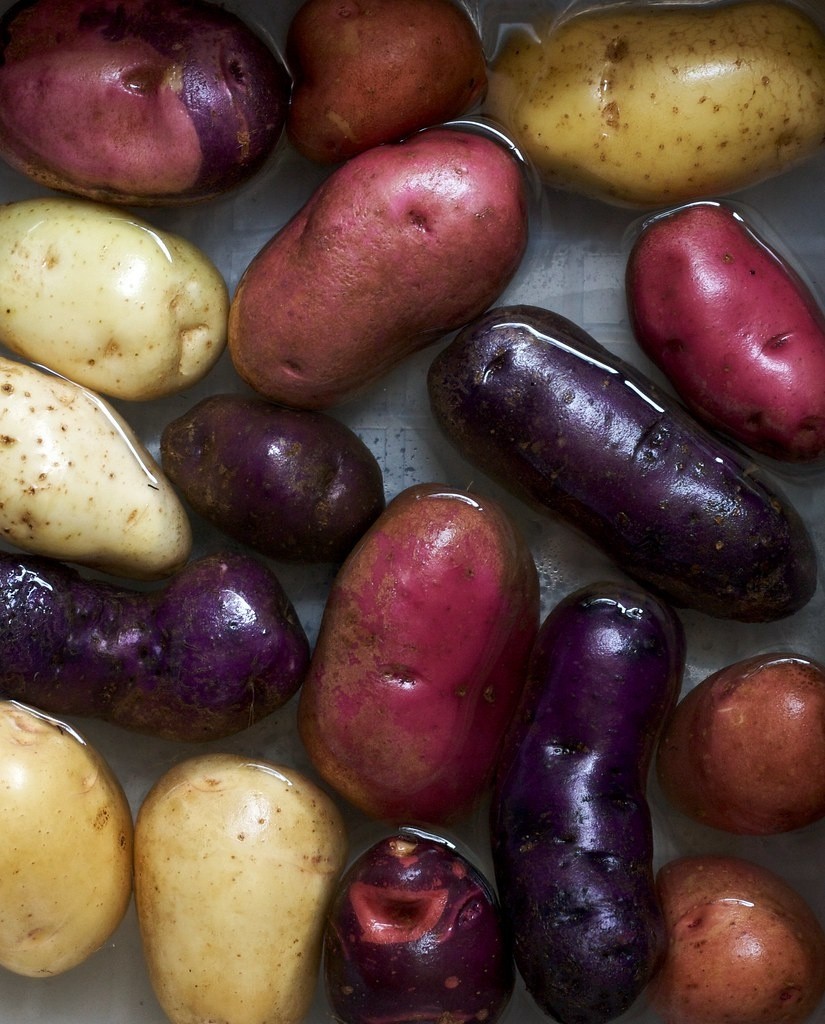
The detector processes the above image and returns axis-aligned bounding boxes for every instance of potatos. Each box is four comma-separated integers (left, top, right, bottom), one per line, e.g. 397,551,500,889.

1,0,823,1024
